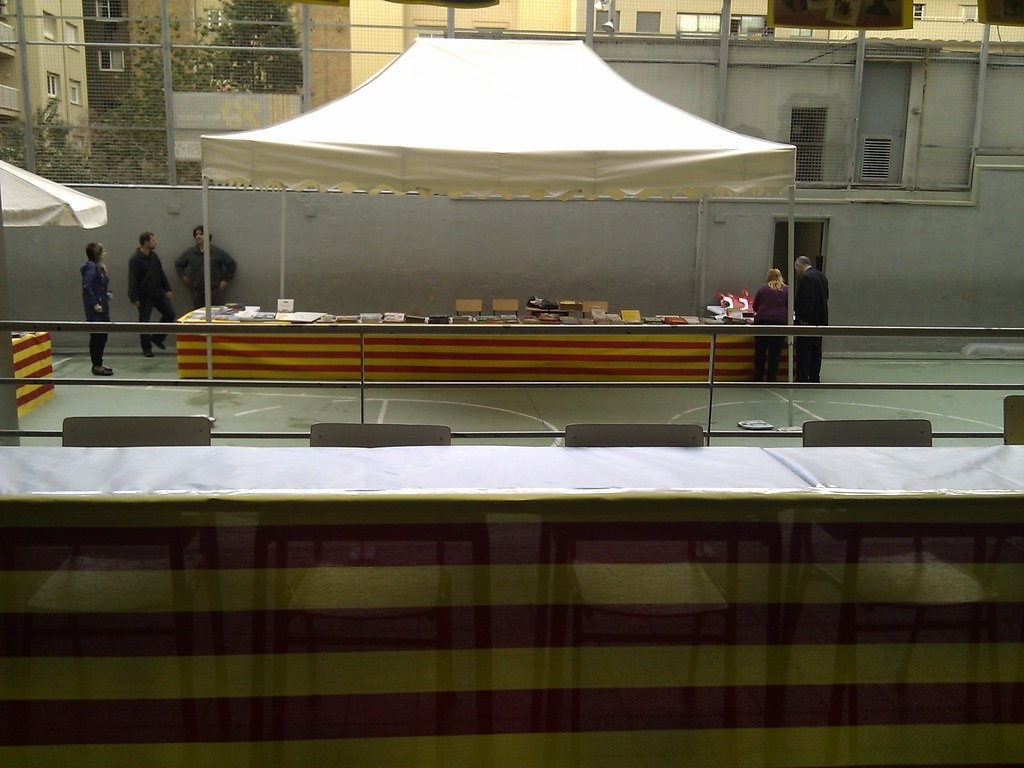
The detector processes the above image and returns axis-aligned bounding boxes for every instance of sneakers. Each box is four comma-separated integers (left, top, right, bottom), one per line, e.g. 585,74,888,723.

795,376,809,382
753,377,764,381
808,376,820,383
151,337,165,349
766,378,776,381
143,347,153,357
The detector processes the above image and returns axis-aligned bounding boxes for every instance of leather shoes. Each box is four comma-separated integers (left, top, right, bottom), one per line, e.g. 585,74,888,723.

92,367,113,376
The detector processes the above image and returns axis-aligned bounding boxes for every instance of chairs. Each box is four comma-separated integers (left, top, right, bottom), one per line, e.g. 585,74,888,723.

456,300,482,315
492,299,519,317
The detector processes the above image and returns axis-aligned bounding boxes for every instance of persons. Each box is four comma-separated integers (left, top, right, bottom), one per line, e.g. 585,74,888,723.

794,256,829,383
79,242,114,376
176,226,236,308
128,233,174,357
752,269,788,382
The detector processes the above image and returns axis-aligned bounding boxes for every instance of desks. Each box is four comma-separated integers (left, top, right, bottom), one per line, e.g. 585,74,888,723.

0,442,1024,768
175,303,796,387
12,332,55,417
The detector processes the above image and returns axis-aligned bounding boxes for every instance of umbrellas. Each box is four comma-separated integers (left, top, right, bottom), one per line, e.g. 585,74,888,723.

0,160,109,229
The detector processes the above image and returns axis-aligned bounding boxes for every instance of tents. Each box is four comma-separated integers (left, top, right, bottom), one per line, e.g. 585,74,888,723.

201,36,796,429
